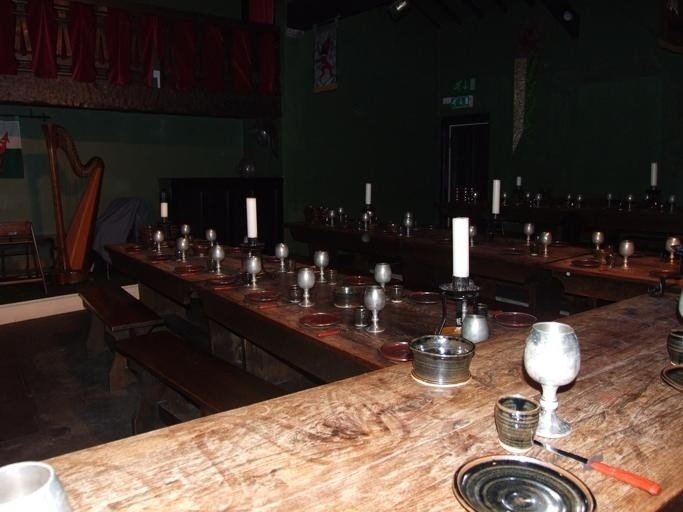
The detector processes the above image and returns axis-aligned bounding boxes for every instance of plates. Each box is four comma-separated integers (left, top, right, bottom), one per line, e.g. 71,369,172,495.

432,232,452,241
572,259,599,269
451,452,599,511
300,312,343,326
377,342,411,363
410,289,443,305
501,247,524,255
493,311,538,326
127,242,152,256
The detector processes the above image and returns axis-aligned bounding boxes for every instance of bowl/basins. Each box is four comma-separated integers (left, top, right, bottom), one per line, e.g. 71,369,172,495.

409,334,475,385
334,275,368,307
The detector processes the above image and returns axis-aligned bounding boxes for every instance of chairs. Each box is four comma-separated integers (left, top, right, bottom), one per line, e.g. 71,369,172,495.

0,220,49,298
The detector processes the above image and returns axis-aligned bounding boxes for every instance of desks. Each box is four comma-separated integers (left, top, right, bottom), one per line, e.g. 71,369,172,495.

103,192,681,390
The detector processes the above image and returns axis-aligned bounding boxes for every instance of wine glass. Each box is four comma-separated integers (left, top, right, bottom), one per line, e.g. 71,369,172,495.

593,231,605,256
500,192,679,214
521,223,538,247
525,322,582,436
619,239,635,269
306,201,421,238
468,223,480,247
664,236,680,270
150,225,328,308
541,230,555,256
364,262,392,332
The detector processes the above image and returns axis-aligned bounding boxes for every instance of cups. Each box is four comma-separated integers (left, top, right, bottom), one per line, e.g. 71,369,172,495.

460,303,491,343
391,283,405,299
328,267,339,285
352,307,370,329
0,460,71,512
666,328,682,363
492,394,538,446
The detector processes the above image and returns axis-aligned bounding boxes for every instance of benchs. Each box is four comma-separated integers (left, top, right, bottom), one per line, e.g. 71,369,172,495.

73,280,166,356
109,327,293,435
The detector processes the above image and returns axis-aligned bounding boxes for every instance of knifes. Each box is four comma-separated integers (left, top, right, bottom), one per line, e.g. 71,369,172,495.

534,438,660,499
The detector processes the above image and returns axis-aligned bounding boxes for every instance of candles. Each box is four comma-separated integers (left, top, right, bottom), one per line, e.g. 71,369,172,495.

159,202,169,219
489,178,504,225
451,217,470,277
515,174,522,199
362,180,376,214
647,160,659,201
246,197,257,237
